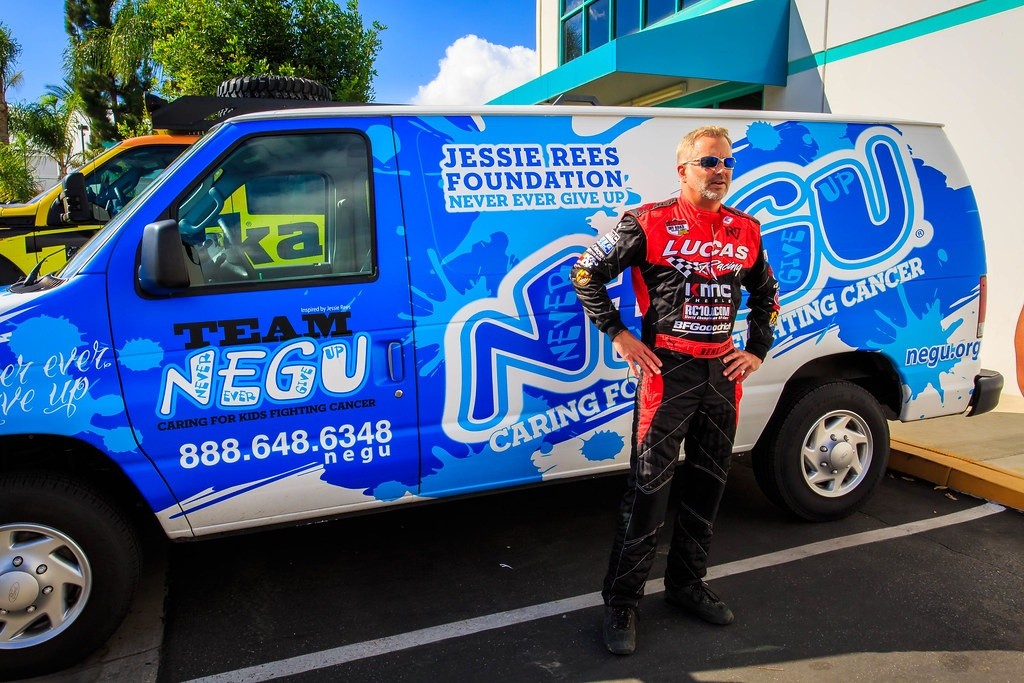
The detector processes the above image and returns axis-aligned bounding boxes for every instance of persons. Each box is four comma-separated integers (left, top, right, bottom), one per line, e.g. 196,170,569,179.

571,125,780,655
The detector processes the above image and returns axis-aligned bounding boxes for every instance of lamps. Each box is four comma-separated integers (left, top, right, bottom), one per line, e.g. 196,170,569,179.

632,81,687,106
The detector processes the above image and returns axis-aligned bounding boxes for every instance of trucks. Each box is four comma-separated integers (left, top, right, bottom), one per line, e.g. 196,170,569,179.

0,106,1002,682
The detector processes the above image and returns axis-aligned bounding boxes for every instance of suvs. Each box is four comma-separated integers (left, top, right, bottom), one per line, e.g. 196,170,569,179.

0,77,337,287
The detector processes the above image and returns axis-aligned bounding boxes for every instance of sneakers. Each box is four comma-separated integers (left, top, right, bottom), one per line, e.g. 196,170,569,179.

664,576,736,627
600,601,640,656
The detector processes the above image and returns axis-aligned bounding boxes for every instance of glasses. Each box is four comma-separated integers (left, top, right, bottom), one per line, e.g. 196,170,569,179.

680,155,737,170
